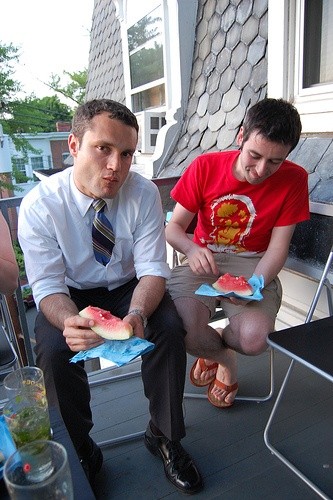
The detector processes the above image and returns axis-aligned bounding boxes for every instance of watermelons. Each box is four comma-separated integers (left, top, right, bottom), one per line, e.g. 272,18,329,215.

212,272,255,296
79,305,132,340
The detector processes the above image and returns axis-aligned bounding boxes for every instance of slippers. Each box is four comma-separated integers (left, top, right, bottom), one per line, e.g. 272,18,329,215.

208,379,238,408
190,357,218,387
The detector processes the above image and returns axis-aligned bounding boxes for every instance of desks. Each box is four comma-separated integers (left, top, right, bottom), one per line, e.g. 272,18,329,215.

0,405,98,500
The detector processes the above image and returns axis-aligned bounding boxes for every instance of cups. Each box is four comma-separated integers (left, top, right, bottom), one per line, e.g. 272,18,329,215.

3,366,46,410
3,439,74,500
3,392,56,482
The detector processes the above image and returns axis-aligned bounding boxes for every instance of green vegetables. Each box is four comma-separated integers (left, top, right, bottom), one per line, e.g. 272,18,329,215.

11,408,51,455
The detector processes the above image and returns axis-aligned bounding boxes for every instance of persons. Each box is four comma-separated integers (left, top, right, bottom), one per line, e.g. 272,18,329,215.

15,100,203,500
164,98,311,407
0,211,19,295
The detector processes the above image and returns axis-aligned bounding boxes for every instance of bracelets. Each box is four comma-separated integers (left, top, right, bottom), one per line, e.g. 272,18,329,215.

128,309,148,329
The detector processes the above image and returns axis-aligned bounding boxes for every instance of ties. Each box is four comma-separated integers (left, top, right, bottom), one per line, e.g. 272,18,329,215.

92,199,115,266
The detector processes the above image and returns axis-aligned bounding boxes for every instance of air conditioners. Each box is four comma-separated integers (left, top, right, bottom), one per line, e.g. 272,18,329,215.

132,110,170,156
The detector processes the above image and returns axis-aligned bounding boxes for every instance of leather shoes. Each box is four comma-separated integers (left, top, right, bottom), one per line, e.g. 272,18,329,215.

144,422,203,494
70,440,103,500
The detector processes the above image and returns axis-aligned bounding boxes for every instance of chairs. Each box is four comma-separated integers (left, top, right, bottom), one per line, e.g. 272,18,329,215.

0,262,39,384
57,363,187,451
261,241,333,500
170,214,277,405
